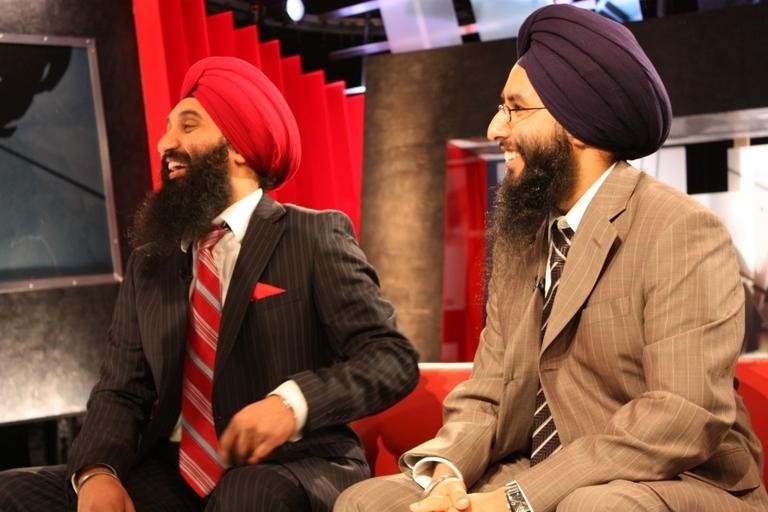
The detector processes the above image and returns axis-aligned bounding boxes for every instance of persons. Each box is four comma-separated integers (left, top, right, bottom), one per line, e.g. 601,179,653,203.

1,51,420,510
328,2,764,511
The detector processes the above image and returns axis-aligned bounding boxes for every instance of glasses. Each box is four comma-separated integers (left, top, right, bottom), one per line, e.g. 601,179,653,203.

495,104,547,124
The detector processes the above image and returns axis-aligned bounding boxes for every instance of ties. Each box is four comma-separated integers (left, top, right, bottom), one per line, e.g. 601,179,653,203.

177,221,232,500
530,217,575,467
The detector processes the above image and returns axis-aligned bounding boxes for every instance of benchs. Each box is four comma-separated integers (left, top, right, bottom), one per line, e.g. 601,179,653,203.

347,351,768,512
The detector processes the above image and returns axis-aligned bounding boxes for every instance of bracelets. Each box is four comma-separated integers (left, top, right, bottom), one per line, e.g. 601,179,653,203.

423,474,454,496
78,468,119,488
503,478,531,511
